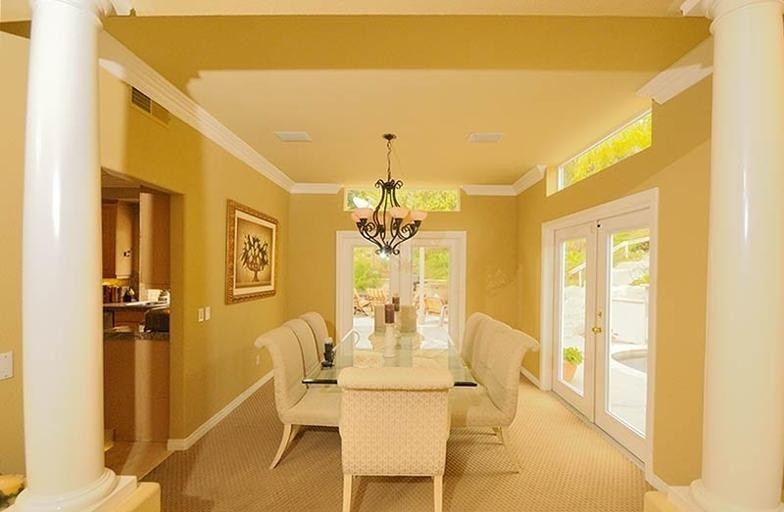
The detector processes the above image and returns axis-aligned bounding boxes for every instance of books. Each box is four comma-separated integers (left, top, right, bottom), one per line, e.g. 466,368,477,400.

115,329,133,334
105,286,111,302
126,300,168,308
111,287,115,302
112,325,130,329
120,286,130,303
115,287,120,302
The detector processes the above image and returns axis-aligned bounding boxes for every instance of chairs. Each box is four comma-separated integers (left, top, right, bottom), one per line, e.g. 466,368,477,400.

334,364,454,512
449,328,539,473
299,312,333,361
284,319,338,384
468,318,511,392
458,312,491,370
353,288,448,321
254,324,340,470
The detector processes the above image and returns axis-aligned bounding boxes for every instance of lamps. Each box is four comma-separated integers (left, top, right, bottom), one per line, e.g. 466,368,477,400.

349,133,427,260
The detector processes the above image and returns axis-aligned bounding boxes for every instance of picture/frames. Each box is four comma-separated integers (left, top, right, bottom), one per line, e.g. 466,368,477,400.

226,197,276,305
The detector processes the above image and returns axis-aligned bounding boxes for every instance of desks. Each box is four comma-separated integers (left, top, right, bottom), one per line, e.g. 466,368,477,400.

330,324,478,388
